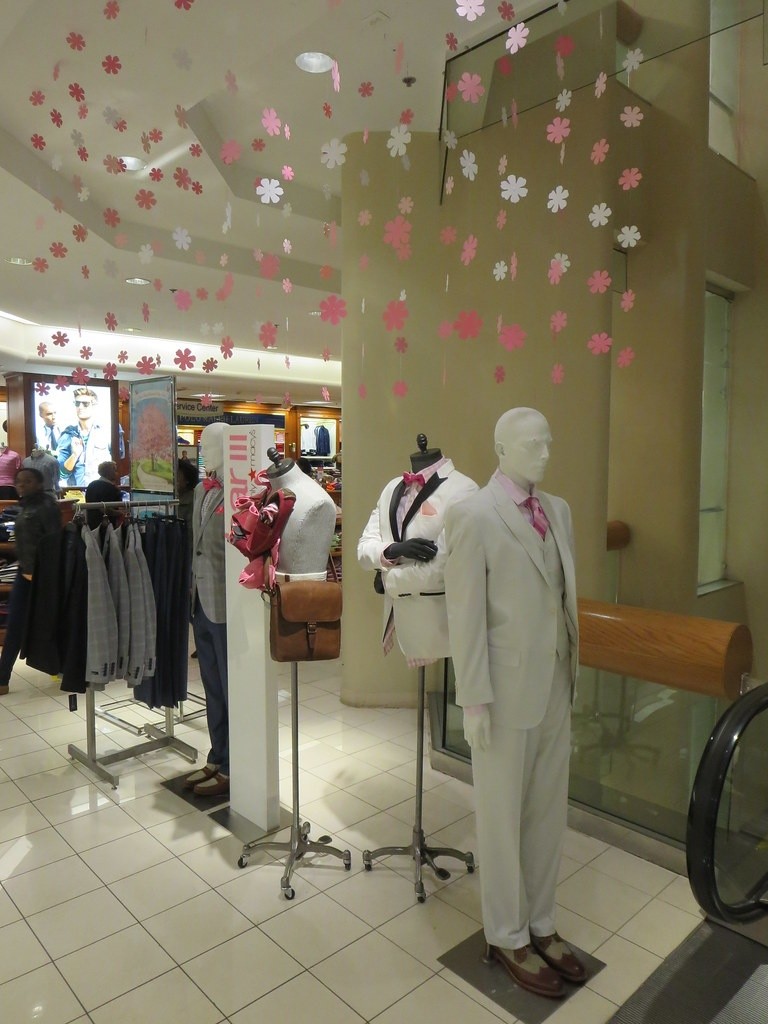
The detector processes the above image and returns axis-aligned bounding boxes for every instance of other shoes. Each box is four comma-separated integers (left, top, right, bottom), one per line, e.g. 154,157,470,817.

245,488,296,554
185,766,219,788
193,774,230,794
0,686,9,695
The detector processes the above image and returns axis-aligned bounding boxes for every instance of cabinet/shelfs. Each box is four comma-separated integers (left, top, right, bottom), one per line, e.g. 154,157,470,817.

0,500,23,593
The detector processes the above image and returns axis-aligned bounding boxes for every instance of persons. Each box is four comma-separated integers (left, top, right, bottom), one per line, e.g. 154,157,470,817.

36,401,64,453
0,442,21,500
56,388,111,487
21,448,63,500
186,421,231,794
257,456,336,581
85,461,122,531
356,448,481,668
0,469,60,697
443,408,588,999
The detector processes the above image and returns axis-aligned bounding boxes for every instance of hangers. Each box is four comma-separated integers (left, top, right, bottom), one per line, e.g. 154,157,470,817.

64,500,185,533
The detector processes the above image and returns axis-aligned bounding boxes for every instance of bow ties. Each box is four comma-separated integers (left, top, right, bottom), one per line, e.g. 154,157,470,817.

203,478,222,492
403,471,425,487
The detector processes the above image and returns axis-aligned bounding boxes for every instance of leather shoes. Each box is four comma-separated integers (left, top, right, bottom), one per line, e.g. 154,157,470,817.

493,942,567,996
529,922,588,982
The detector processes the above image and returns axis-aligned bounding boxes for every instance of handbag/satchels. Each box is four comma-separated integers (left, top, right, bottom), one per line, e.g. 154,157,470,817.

270,581,343,662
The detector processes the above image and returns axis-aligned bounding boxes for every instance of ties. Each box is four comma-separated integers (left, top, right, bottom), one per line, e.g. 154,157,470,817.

50,426,58,451
525,497,549,542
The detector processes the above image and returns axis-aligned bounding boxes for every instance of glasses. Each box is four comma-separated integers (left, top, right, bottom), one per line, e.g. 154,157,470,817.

75,401,90,407
